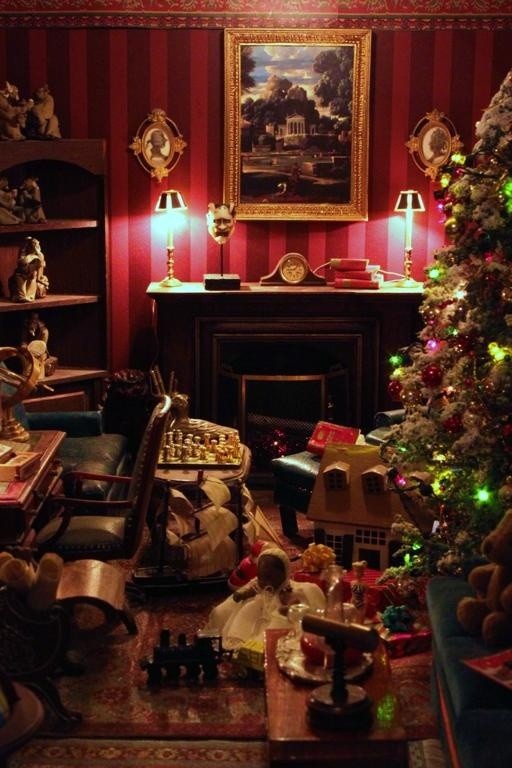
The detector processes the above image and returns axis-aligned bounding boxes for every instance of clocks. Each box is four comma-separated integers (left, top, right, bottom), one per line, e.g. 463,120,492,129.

259,252,326,286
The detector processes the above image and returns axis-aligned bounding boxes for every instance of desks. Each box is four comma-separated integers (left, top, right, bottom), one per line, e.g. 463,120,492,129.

0,425,68,556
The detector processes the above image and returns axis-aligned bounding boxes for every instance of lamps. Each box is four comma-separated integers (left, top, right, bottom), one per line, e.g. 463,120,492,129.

394,189,427,288
154,189,189,288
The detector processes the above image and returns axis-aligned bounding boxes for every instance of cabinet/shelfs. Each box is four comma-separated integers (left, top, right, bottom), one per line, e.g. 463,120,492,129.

2,135,112,419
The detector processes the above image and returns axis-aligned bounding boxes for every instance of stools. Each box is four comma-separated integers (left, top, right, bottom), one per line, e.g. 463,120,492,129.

268,450,362,538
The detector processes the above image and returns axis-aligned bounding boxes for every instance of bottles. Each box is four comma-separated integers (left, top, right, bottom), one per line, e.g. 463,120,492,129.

325,563,348,626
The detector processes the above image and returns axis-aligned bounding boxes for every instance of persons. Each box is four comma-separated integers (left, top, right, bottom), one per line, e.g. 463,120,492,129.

0,175,45,226
0,83,63,142
207,201,237,244
198,541,371,678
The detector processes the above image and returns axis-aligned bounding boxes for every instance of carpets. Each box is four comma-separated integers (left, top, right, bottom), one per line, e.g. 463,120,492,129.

0,740,447,768
33,488,439,738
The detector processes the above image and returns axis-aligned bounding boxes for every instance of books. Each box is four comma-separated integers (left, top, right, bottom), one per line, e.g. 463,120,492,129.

306,420,366,459
329,257,384,289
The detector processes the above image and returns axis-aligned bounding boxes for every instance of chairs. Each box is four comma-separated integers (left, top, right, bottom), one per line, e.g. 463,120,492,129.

22,392,174,638
2,360,132,514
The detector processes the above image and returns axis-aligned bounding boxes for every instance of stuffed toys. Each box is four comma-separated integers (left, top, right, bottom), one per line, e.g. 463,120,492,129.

456,509,511,649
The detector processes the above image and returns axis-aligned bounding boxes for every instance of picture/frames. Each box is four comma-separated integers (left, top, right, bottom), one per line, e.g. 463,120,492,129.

223,28,370,222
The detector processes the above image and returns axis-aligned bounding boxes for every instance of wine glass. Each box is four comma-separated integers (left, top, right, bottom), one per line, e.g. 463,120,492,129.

287,598,312,651
